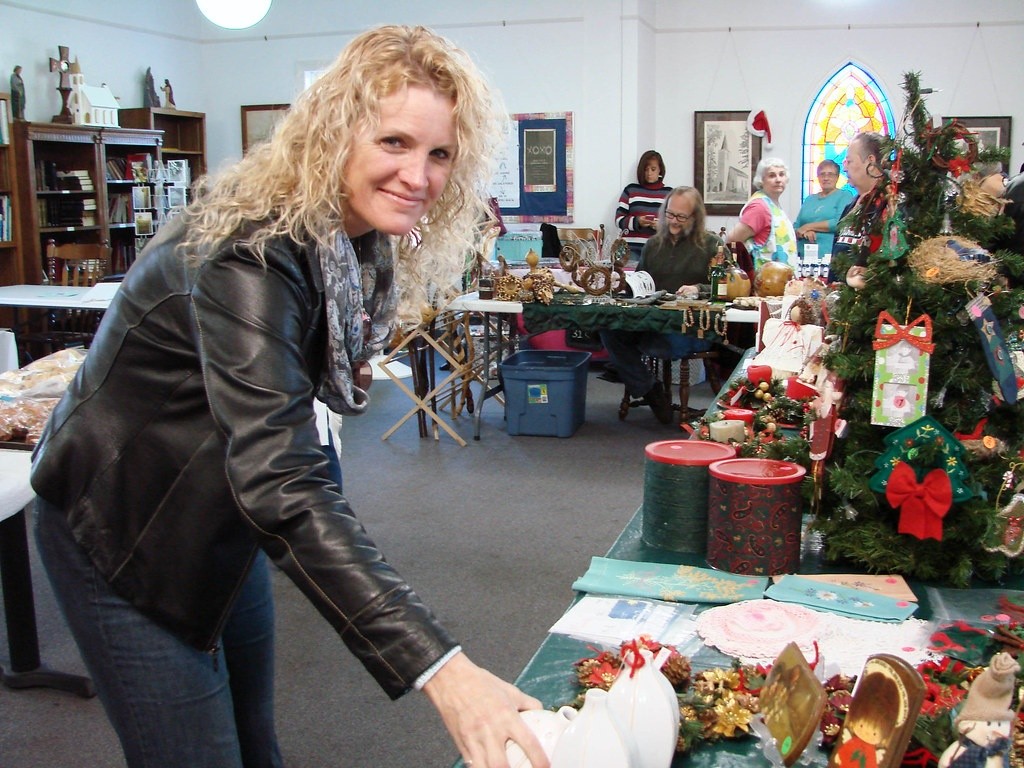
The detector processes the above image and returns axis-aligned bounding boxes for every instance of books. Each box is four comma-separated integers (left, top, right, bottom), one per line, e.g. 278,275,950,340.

0,99,10,145
0,194,13,243
161,157,195,218
35,151,154,285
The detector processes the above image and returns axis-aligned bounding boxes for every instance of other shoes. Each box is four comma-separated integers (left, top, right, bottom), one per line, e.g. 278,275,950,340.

641,380,672,424
602,361,619,375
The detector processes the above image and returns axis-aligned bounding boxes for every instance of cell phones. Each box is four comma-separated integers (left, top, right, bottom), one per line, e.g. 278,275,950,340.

645,215,655,221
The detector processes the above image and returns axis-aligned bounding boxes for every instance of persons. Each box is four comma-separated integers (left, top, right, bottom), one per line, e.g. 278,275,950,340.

795,160,854,266
29,25,551,768
10,66,26,123
723,158,797,272
753,298,814,378
591,150,734,425
828,131,896,283
760,663,801,724
160,78,175,110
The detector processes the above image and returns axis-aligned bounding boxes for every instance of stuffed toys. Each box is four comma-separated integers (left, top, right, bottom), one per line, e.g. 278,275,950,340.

939,651,1020,768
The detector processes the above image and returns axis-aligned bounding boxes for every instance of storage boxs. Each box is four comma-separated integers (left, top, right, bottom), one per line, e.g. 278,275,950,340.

467,324,520,381
498,349,593,437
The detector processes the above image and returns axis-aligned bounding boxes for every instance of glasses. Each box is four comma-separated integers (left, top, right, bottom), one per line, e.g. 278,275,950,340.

664,210,694,222
817,172,837,178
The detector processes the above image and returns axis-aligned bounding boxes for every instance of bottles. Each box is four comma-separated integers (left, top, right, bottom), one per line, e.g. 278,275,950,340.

731,242,740,270
710,245,727,303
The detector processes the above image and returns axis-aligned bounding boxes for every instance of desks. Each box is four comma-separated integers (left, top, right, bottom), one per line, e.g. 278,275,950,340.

445,289,767,441
448,346,1024,768
0,282,122,367
0,344,111,700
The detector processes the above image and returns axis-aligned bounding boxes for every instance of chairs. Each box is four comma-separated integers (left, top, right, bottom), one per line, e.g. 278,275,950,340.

539,221,606,261
727,240,755,297
14,239,110,368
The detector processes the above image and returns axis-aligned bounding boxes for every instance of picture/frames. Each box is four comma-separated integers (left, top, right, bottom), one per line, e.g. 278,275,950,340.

694,110,762,212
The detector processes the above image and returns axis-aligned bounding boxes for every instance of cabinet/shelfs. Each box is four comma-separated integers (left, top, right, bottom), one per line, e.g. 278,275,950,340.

117,108,208,216
13,119,165,362
0,91,29,370
